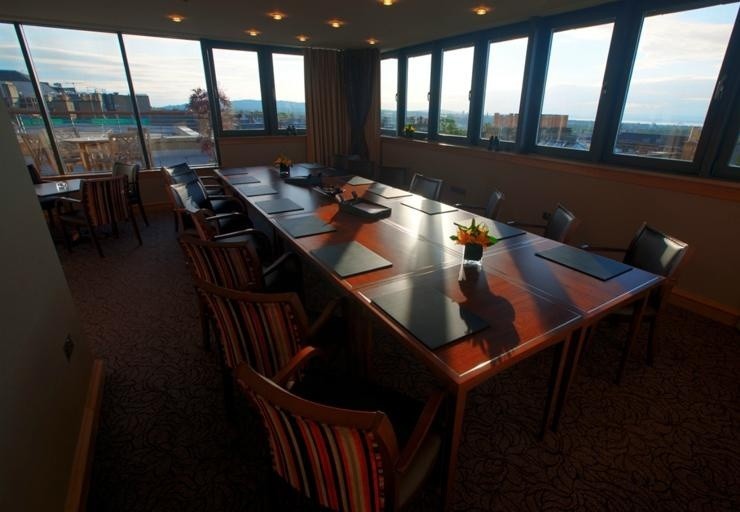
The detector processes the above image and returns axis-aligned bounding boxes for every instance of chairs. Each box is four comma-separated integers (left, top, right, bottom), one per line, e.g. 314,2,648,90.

573,219,691,387
178,234,298,351
505,202,579,244
231,364,450,511
24,162,71,228
187,273,352,405
157,157,247,271
451,185,509,225
103,160,149,236
58,173,145,261
404,170,444,202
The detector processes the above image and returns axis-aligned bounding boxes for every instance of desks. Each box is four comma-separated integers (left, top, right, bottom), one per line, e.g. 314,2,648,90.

29,177,110,256
211,159,672,417
